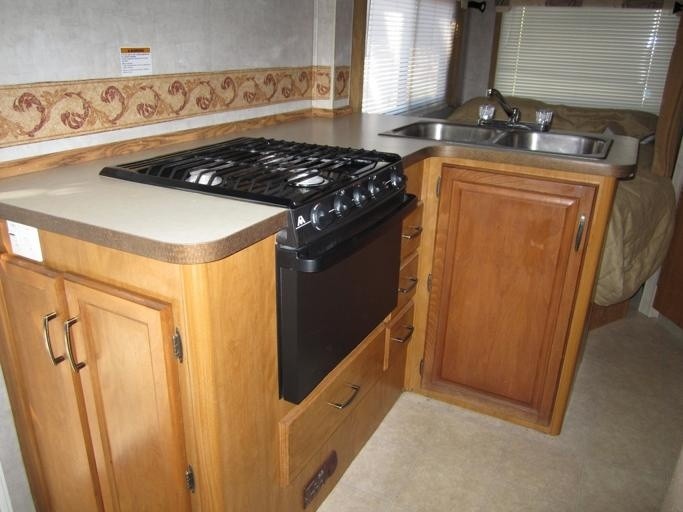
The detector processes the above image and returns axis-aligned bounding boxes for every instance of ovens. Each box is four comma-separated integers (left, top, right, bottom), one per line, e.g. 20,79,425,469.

275,189,418,405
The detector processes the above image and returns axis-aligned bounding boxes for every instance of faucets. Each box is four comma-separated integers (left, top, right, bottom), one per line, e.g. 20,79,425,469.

485,88,522,122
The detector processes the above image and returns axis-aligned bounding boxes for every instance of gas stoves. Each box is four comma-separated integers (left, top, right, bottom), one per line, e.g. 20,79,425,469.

100,136,403,210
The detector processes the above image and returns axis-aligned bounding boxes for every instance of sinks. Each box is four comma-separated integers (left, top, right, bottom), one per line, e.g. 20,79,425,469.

498,132,606,153
395,122,497,142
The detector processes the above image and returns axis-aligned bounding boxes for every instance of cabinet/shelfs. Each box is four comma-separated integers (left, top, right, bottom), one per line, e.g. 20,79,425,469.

280,322,390,511
1,219,283,511
419,158,600,428
382,161,427,411
